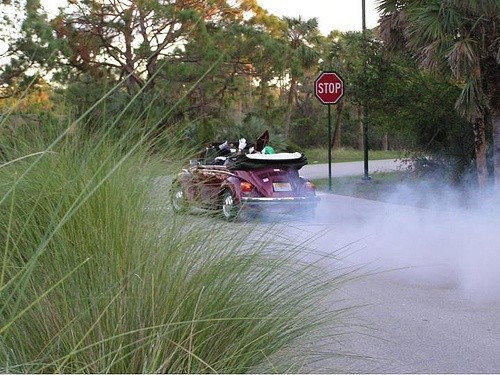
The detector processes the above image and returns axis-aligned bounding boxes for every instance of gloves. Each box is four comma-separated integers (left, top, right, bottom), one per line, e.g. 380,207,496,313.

238,138,247,150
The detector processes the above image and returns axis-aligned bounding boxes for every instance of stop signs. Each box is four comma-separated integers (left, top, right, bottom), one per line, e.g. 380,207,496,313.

314,71,345,105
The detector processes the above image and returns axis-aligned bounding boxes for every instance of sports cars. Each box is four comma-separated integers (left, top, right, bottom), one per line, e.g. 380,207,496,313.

170,141,321,222
